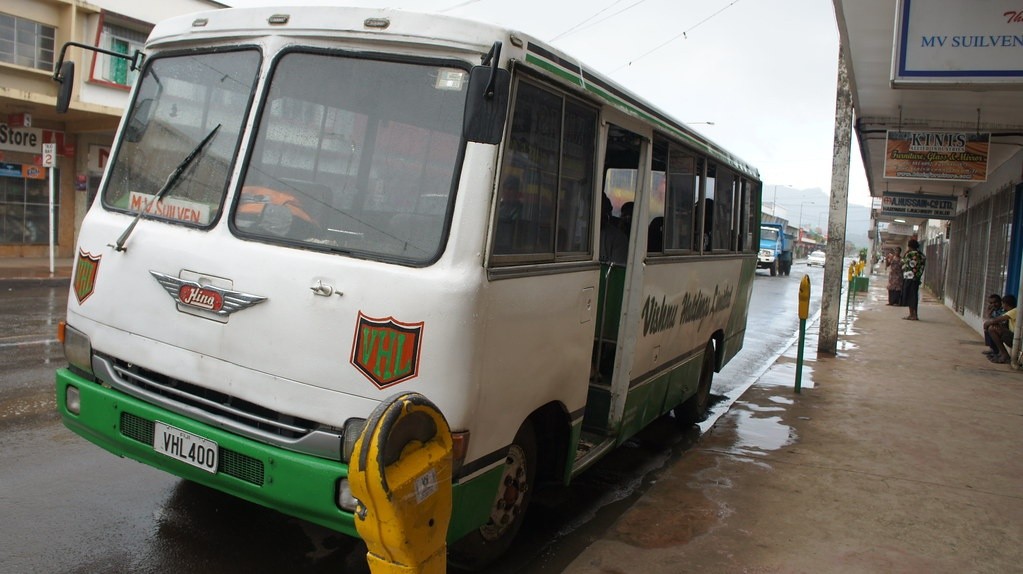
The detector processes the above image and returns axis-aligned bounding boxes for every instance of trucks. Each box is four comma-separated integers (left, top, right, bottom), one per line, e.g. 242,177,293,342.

754,223,797,276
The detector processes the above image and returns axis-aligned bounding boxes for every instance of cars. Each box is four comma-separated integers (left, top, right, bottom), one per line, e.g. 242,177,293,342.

849,252,860,258
807,252,826,268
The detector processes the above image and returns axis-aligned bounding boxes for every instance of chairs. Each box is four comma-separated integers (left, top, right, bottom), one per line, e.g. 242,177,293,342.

273,177,334,245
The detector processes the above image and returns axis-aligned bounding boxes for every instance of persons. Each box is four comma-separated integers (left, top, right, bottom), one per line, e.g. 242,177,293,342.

899,239,927,321
983,294,1018,364
885,247,904,306
497,174,715,265
981,294,1009,361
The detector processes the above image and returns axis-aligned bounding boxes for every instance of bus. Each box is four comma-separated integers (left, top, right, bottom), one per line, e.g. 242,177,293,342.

50,12,762,554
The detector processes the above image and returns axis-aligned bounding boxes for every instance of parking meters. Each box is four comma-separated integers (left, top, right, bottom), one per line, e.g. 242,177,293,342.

794,274,811,392
845,266,853,310
853,261,864,297
